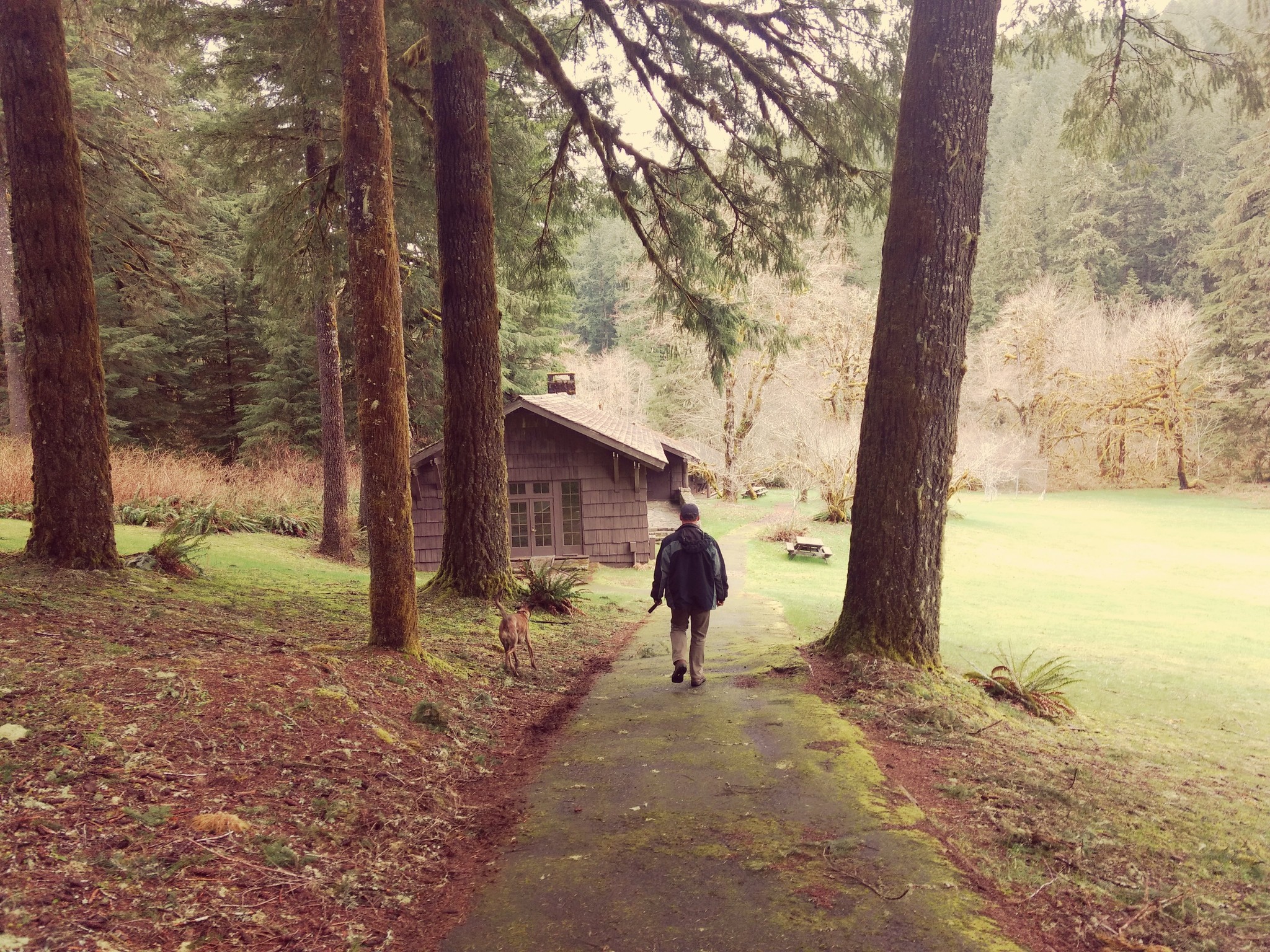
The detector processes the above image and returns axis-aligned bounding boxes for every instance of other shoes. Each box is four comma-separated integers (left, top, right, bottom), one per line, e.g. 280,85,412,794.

671,660,687,683
691,674,706,686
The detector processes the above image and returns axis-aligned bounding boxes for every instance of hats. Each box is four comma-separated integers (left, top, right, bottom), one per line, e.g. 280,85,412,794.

680,503,699,515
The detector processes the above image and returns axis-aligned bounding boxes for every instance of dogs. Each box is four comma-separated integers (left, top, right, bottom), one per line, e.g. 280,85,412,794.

495,589,537,677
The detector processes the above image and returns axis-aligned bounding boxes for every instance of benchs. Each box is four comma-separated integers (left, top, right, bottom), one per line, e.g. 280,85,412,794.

786,542,795,552
756,490,768,495
822,546,833,556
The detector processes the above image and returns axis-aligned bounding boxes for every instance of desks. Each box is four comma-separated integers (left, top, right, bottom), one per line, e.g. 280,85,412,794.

747,486,766,497
788,536,829,565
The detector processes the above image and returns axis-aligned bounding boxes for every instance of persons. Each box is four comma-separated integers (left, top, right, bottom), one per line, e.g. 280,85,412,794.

651,503,729,686
